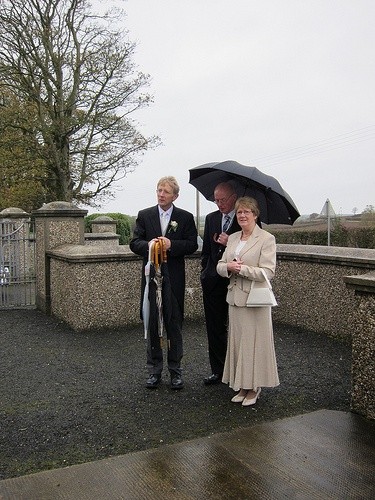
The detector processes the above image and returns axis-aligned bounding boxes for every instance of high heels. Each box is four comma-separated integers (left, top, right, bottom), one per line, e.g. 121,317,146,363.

242,388,261,406
231,394,245,402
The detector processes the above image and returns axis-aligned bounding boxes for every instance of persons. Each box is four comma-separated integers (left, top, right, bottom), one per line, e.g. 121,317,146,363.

129,176,197,390
201,184,264,386
216,196,281,407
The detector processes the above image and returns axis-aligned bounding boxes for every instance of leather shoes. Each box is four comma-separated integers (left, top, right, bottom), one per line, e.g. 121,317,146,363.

146,373,162,388
170,372,184,389
203,374,222,383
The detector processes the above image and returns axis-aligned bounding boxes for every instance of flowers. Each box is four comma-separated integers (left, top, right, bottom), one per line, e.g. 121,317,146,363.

168,220,178,233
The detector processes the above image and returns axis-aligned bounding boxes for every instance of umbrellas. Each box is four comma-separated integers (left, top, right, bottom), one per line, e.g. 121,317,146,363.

143,238,172,351
188,160,301,243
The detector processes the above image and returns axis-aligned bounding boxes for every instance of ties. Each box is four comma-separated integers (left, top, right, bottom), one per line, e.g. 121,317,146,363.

223,215,231,233
160,212,168,238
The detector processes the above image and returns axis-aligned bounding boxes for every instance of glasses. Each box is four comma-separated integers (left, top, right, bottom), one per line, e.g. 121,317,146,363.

236,209,254,215
213,192,235,204
156,189,172,195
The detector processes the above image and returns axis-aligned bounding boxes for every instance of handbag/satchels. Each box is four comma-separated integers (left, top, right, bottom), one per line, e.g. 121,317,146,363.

246,268,273,308
